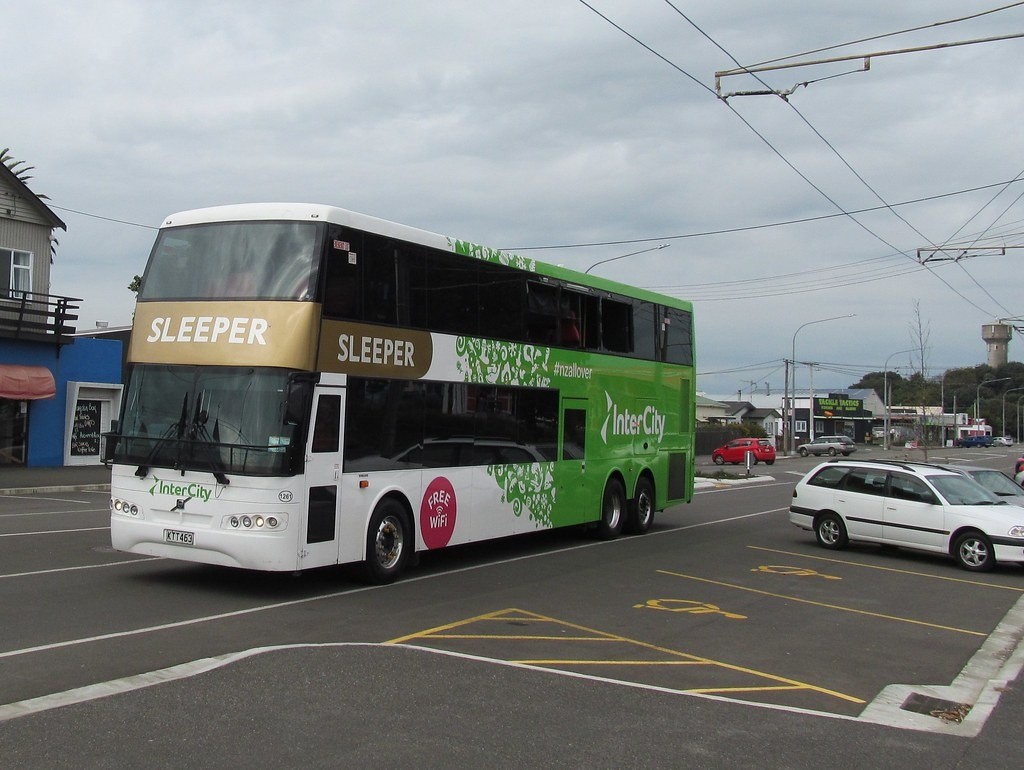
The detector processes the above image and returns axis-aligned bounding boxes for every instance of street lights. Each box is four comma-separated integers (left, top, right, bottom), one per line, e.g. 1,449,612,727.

790,313,858,456
976,378,1011,436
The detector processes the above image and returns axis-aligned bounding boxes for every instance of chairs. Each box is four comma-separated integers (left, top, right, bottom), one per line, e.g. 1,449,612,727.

843,470,943,505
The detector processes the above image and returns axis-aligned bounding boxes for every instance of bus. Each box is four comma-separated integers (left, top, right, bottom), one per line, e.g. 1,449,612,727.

99,201,696,586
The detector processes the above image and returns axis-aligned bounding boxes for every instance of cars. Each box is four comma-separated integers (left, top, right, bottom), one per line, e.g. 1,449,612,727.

936,461,1023,509
785,458,1024,570
992,437,1014,447
956,435,994,448
872,427,884,438
712,438,775,465
796,434,858,457
